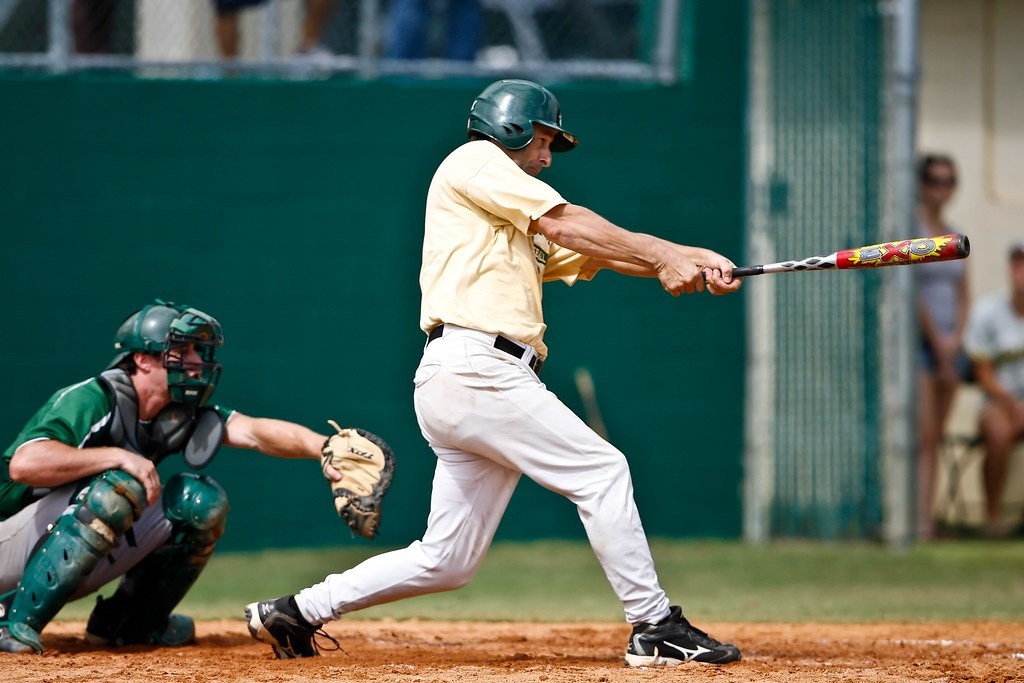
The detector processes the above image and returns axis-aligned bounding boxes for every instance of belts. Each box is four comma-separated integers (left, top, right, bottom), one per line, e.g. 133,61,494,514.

426,324,543,375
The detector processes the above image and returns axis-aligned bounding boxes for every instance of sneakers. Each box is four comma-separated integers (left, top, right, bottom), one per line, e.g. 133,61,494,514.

244,595,349,659
0,626,33,653
625,605,741,667
84,594,195,646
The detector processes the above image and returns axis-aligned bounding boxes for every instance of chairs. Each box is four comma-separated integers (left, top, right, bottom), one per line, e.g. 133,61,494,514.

936,347,985,534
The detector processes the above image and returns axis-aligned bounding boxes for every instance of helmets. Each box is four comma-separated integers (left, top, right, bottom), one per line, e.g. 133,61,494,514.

467,79,577,152
105,302,224,370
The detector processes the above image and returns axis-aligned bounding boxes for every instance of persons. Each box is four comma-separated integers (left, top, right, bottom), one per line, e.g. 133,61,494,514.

0,300,342,654
911,152,1024,545
246,80,743,672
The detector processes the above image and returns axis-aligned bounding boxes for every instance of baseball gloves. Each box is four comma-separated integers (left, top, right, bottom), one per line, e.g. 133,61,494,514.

320,418,398,541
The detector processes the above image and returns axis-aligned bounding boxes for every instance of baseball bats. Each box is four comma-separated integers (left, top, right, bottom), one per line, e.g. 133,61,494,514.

658,233,971,290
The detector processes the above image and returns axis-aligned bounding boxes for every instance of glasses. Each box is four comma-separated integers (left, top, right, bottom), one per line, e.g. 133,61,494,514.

924,173,958,188
